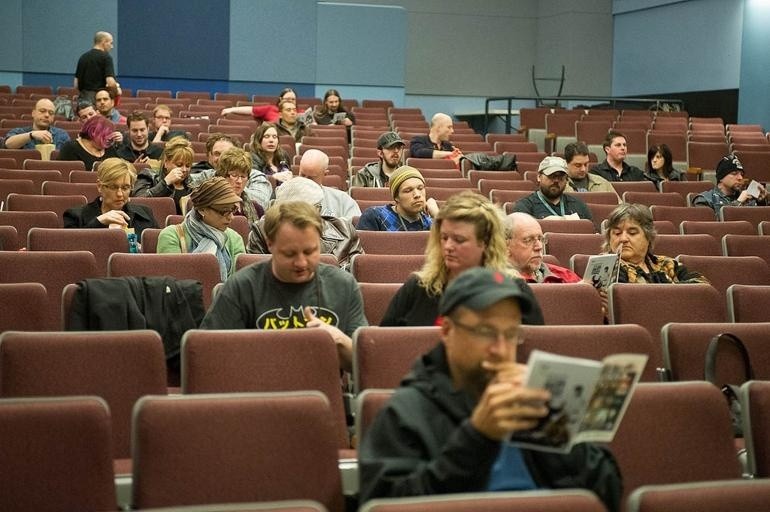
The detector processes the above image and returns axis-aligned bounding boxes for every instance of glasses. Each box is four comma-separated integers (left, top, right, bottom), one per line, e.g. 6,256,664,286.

103,184,131,192
455,321,526,345
512,236,548,246
544,175,567,181
208,207,239,215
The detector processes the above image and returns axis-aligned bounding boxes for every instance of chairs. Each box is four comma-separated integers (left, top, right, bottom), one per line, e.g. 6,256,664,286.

1,64,769,510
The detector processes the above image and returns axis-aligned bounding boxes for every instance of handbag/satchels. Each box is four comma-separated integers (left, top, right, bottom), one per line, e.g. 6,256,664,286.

705,332,753,436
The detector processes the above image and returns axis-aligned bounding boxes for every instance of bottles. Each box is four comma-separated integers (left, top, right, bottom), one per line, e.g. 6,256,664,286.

127,234,138,253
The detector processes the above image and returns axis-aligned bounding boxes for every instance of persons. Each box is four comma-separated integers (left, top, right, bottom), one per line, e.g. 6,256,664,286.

73,31,122,110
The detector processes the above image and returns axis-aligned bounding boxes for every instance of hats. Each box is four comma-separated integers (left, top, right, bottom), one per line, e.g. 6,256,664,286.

538,156,569,176
191,175,244,209
388,166,426,198
437,266,535,316
275,177,324,206
376,132,406,149
715,155,745,183
